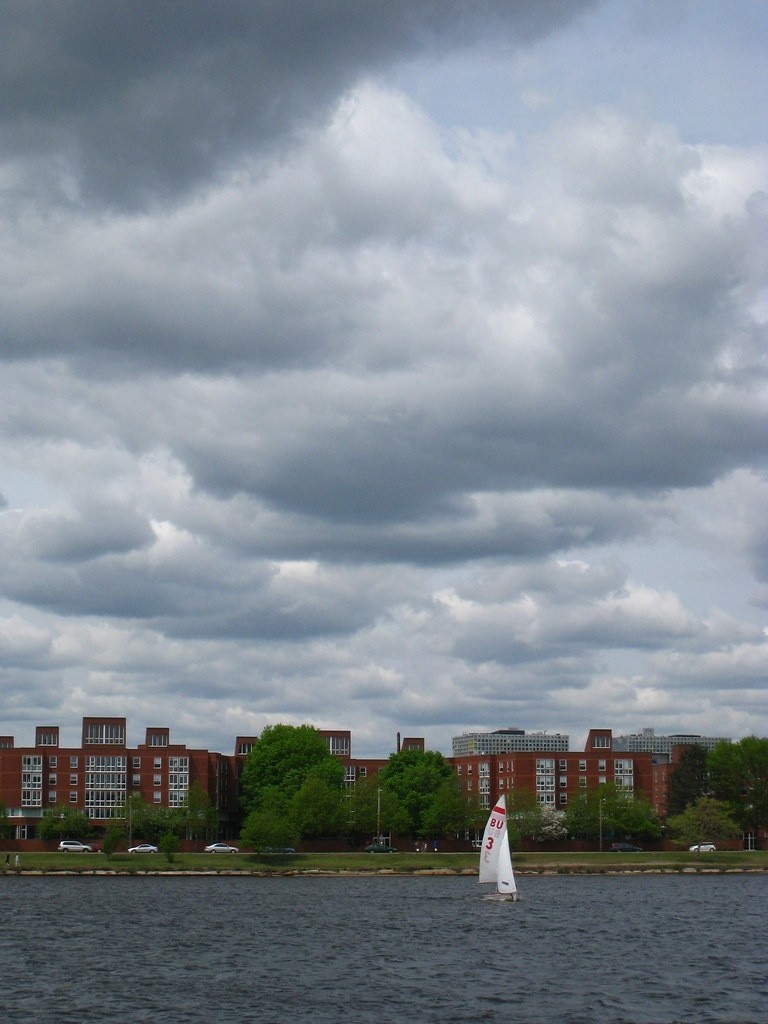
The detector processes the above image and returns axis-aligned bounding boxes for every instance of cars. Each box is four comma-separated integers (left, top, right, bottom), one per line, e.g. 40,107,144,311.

205,843,239,853
128,844,158,853
255,846,295,853
57,841,91,853
609,842,644,853
688,842,716,851
363,843,397,853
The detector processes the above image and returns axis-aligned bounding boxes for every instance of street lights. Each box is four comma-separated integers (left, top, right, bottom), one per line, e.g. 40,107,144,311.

599,798,606,851
377,787,383,843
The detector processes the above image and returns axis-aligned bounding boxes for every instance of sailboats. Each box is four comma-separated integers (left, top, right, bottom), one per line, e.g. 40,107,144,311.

479,793,518,902
